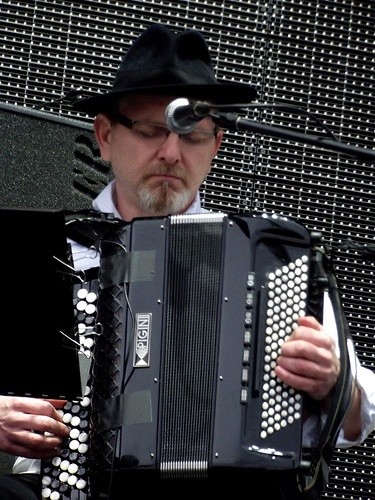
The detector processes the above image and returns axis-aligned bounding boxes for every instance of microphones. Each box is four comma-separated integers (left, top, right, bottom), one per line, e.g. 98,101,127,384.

165,97,210,134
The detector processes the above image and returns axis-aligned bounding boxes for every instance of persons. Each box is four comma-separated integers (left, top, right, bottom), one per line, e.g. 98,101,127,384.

0,22,375,500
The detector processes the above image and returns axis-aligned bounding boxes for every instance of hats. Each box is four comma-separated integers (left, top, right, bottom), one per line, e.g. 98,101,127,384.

68,24,259,114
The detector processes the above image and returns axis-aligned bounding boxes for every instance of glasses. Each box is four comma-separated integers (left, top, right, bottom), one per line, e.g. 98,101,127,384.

113,115,218,148
